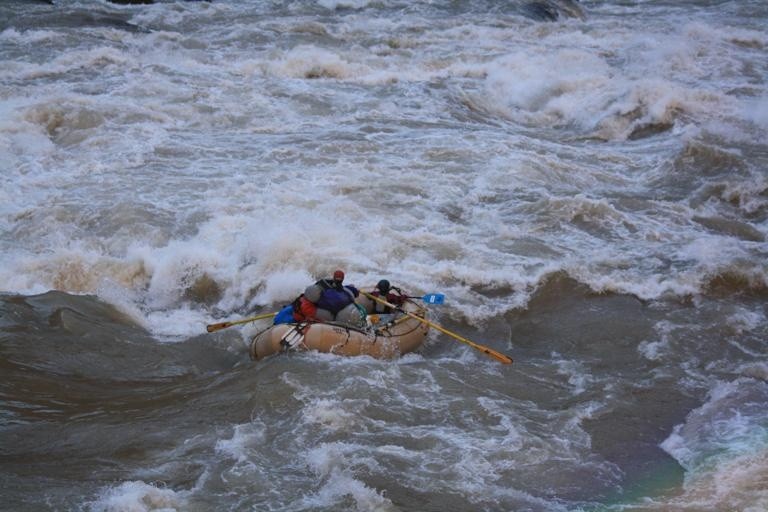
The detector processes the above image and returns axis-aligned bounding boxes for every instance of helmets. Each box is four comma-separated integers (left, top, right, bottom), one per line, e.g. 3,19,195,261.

304,285,321,304
378,279,390,292
334,271,344,281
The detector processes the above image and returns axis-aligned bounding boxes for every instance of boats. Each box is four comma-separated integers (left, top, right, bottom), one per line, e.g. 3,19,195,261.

249,292,430,361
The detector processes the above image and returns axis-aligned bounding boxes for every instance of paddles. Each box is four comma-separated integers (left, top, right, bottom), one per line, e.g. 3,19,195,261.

208,311,279,332
405,294,445,304
357,290,513,366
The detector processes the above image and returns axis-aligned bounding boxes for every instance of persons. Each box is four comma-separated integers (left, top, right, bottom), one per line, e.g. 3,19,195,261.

364,278,409,314
289,283,323,324
314,270,347,291
